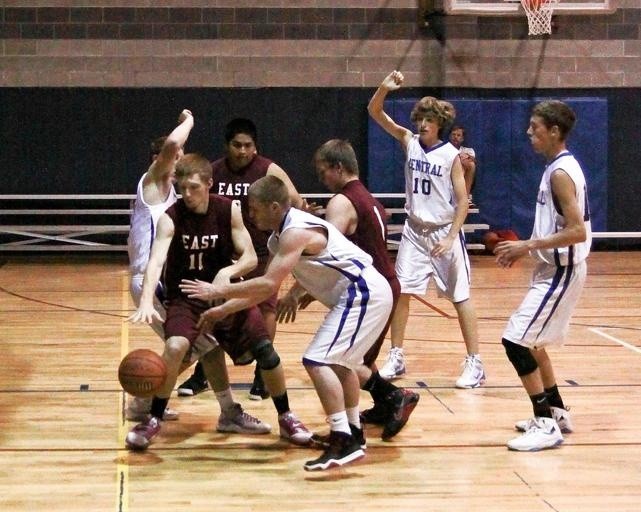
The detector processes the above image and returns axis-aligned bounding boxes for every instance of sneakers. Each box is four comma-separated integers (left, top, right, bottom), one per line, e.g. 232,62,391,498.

380,387,421,442
278,413,313,444
216,404,271,435
249,387,270,400
124,397,180,422
310,423,367,450
358,406,390,423
454,354,487,390
506,417,564,452
378,347,407,380
126,417,161,449
514,406,575,434
177,372,208,396
303,431,365,472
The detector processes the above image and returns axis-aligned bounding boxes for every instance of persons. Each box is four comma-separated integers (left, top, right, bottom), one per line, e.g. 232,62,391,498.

364,71,488,390
179,174,394,472
122,152,314,450
175,116,308,402
123,107,272,435
493,101,593,453
449,123,478,207
274,138,421,442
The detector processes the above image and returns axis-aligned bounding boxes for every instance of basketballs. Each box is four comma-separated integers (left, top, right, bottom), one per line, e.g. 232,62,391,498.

119,350,167,397
